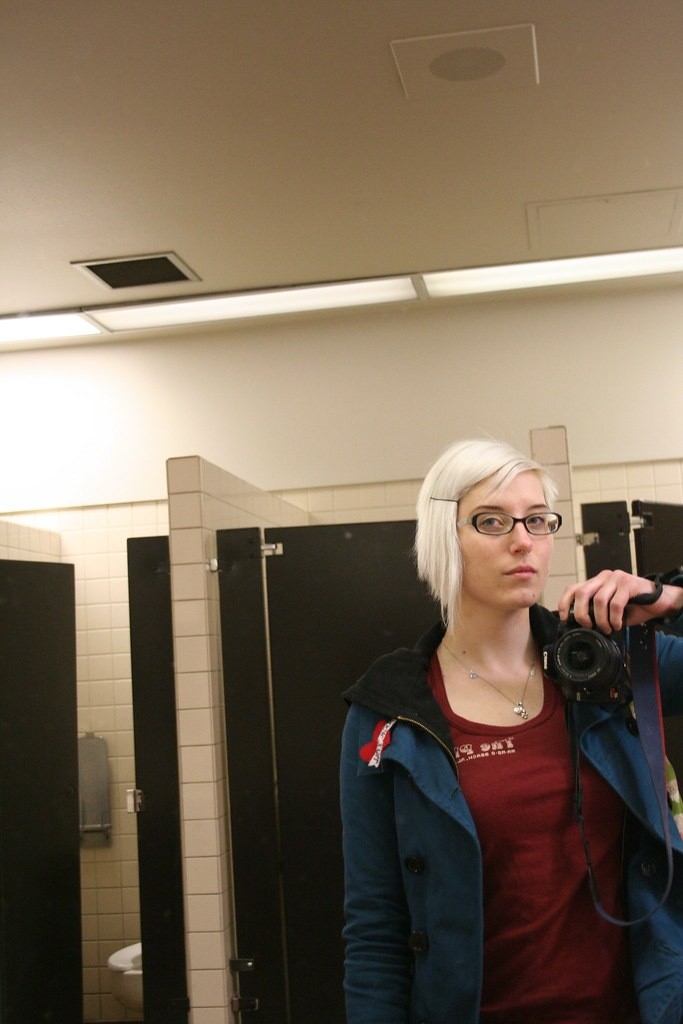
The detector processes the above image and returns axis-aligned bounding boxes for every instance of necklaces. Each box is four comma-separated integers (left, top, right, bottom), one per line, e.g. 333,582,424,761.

442,639,534,717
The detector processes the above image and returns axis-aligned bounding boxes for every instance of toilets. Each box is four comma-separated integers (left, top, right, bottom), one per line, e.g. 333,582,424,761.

107,942,144,1011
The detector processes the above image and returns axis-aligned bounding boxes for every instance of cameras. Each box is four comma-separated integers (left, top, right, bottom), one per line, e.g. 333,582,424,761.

542,605,630,703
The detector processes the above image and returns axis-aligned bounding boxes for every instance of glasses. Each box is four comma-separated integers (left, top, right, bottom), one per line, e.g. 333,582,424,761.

456,512,562,535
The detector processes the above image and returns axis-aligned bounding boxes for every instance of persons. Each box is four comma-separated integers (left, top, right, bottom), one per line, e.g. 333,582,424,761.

338,436,682,1024
